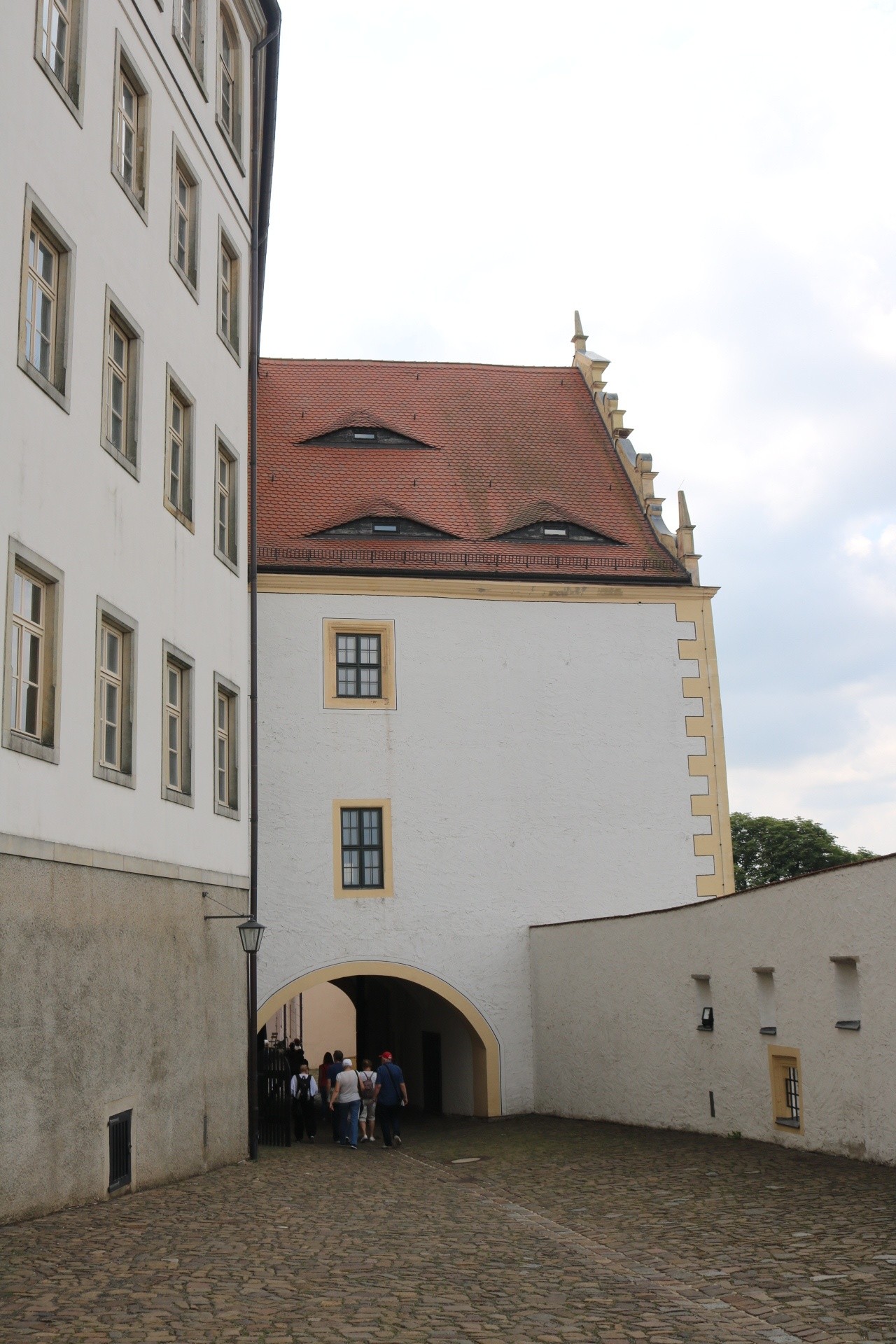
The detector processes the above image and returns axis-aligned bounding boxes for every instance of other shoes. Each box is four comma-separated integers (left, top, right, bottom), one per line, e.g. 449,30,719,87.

351,1144,359,1150
296,1137,302,1142
381,1145,392,1150
369,1137,375,1143
360,1134,368,1143
394,1135,402,1145
309,1134,314,1143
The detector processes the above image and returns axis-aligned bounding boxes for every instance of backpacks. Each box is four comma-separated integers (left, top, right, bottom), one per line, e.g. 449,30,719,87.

361,1072,374,1098
295,1074,312,1102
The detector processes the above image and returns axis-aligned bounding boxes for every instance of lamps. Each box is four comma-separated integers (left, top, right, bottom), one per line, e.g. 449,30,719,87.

701,1006,713,1030
201,890,266,957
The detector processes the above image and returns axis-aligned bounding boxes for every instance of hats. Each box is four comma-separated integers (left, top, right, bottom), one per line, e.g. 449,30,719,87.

342,1059,352,1067
377,1051,392,1059
293,1038,300,1044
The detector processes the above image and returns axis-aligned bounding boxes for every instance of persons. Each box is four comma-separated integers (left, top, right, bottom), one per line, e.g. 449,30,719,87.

329,1059,365,1149
359,1059,378,1142
291,1065,319,1143
318,1050,351,1143
369,1052,408,1149
283,1039,309,1100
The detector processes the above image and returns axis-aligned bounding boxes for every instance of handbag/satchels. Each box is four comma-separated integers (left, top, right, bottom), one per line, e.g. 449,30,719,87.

401,1098,409,1109
